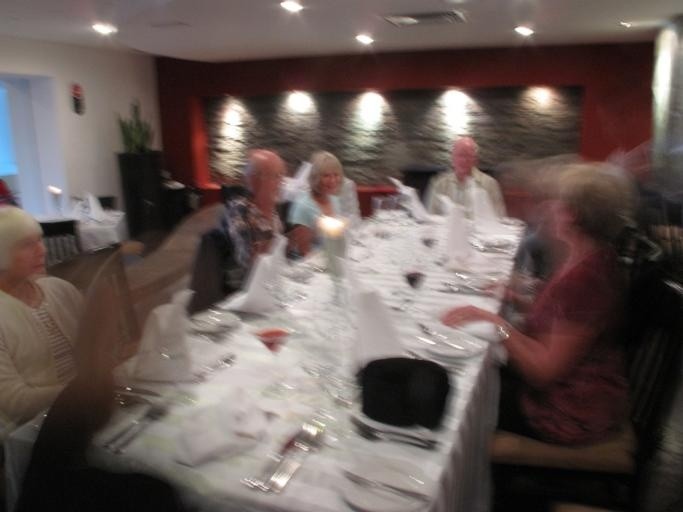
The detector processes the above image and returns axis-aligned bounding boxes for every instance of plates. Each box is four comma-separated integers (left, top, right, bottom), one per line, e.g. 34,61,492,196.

109,213,527,512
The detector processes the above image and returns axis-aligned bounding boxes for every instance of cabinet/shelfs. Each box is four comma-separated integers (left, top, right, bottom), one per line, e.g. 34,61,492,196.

114,147,162,227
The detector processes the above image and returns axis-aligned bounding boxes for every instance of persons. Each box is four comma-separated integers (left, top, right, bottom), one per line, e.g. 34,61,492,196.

284,149,344,237
0,201,127,445
437,159,641,447
182,148,309,319
420,136,509,219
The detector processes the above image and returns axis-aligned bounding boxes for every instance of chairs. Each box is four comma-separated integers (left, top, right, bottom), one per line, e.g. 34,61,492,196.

1,202,683,512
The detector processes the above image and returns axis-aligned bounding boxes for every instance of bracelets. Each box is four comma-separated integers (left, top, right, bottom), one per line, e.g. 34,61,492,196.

495,320,513,341
